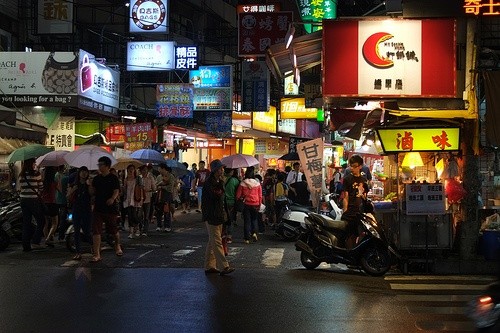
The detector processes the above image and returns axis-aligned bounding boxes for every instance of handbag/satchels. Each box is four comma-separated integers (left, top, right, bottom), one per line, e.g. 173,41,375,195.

37,197,49,212
134,177,145,201
236,199,245,212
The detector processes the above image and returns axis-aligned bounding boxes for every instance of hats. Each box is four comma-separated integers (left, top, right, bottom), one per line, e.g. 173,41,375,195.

211,159,227,175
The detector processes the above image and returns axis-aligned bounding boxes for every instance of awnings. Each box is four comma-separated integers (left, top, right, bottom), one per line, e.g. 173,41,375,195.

264,30,323,88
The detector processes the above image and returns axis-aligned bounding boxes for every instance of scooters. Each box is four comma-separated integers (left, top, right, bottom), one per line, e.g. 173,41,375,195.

294,203,393,276
278,192,350,238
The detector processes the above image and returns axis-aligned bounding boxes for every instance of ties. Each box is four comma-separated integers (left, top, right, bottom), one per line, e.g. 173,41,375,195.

296,172,298,183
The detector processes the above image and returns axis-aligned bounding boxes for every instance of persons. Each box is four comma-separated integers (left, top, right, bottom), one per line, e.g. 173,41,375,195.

123,164,182,238
221,163,306,243
17,157,46,252
329,154,373,269
191,163,197,174
66,166,94,259
86,156,123,262
200,159,235,275
40,165,79,248
193,160,210,213
178,162,194,213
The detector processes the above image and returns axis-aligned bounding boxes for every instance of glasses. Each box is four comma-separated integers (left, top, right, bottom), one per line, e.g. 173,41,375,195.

350,164,359,168
97,163,105,167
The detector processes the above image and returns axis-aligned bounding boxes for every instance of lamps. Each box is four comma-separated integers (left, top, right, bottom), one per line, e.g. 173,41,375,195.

89,57,107,65
401,151,424,169
289,37,323,67
293,59,323,87
284,20,321,48
103,64,119,72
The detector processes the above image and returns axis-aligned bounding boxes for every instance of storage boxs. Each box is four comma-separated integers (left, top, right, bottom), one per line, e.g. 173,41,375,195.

274,194,287,204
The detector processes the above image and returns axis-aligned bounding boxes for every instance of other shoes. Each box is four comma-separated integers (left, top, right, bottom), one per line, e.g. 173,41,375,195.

274,233,283,238
89,255,102,262
44,240,54,246
243,239,248,243
269,224,275,227
253,233,258,240
119,208,202,239
32,242,40,248
219,267,235,276
227,237,231,244
205,268,220,273
73,254,81,259
115,246,123,256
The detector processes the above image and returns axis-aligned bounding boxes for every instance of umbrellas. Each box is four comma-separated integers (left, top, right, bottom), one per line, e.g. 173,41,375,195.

221,153,260,178
64,147,118,180
165,159,183,168
7,144,53,164
36,150,69,166
130,148,163,166
112,156,144,170
277,152,301,166
